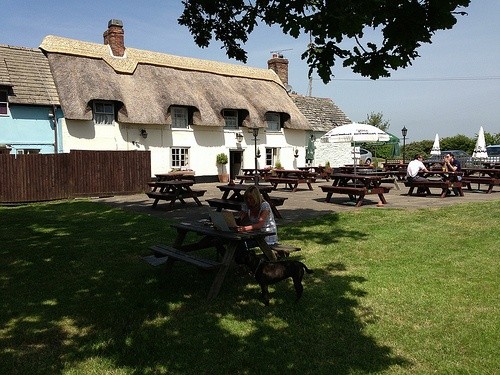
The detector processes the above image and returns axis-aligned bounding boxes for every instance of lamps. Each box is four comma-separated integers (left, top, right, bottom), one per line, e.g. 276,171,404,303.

238,133,244,142
142,129,148,139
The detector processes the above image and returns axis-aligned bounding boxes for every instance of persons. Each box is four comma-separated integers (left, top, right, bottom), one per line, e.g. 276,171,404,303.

366,158,373,166
406,155,431,195
235,186,278,254
440,153,464,197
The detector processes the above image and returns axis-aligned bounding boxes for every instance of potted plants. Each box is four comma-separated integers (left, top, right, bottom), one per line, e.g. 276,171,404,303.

218,153,230,183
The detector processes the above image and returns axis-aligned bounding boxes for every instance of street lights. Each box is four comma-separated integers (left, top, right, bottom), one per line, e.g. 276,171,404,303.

252,122,260,185
400,125,408,164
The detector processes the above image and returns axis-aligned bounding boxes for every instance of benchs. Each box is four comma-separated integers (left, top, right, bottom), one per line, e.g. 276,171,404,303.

429,166,442,170
271,244,301,256
146,190,208,200
147,244,222,272
236,173,271,180
206,196,288,212
387,170,408,176
269,178,316,184
319,183,394,195
462,176,500,185
403,180,471,189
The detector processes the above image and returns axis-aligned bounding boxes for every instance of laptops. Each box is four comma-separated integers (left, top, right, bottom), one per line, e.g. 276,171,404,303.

208,211,236,233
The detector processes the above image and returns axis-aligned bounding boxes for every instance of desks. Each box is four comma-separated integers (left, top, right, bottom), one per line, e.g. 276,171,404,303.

461,168,500,193
381,160,443,180
239,169,272,186
150,181,203,209
409,171,464,198
273,170,314,193
171,220,276,305
324,174,388,207
218,186,282,219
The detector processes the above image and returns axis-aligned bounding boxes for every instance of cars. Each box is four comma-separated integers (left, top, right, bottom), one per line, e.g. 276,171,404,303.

360,148,372,165
487,145,500,162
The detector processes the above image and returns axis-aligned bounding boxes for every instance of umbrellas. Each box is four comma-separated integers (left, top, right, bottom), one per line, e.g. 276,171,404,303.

472,127,488,178
430,133,441,161
320,121,390,201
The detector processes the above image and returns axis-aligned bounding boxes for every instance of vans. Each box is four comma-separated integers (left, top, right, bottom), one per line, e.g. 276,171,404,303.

441,149,472,160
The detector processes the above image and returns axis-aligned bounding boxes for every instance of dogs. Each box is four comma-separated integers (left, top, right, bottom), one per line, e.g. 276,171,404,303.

235,249,315,306
